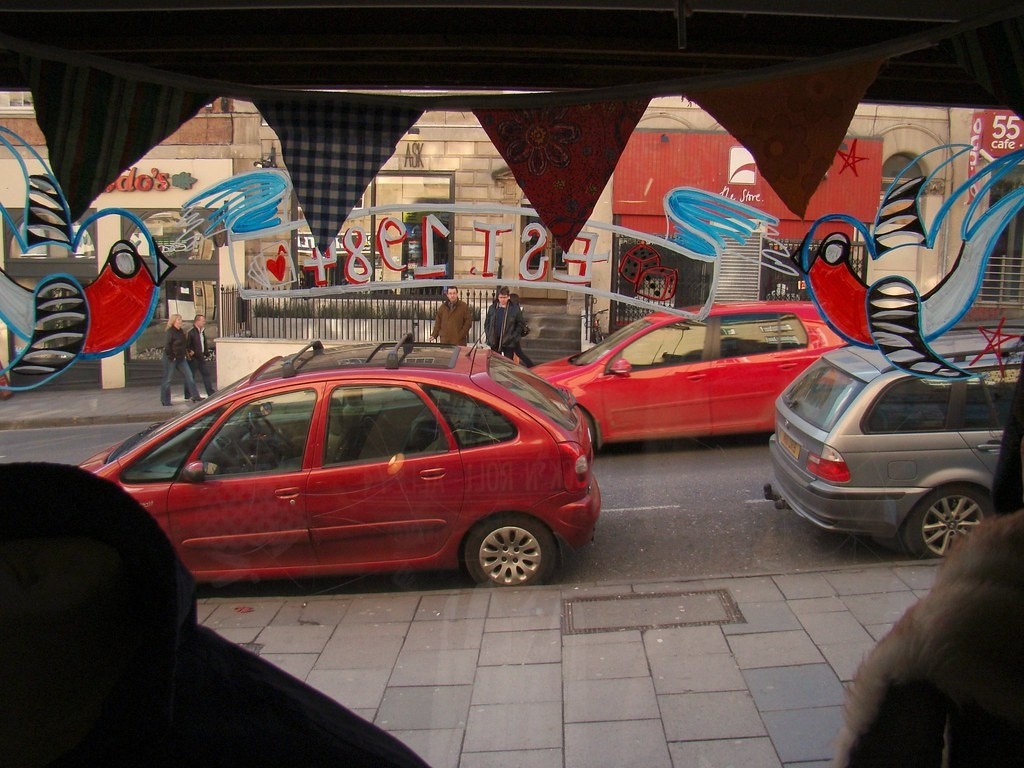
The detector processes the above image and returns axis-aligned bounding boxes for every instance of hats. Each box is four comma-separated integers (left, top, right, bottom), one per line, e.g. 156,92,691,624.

0,537,135,768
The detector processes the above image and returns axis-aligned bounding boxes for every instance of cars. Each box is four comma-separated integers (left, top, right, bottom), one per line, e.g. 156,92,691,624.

403,222,448,262
526,300,854,448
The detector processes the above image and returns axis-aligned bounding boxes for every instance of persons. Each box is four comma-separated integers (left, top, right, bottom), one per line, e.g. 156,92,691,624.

833,360,1024,766
184,315,218,398
161,314,204,406
484,287,533,367
430,286,472,346
0,463,423,767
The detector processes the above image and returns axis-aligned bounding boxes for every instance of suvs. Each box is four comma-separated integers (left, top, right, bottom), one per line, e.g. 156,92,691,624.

763,325,1024,560
75,332,602,587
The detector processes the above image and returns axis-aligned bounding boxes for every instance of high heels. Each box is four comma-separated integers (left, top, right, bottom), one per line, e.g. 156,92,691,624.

161,399,172,406
192,396,205,403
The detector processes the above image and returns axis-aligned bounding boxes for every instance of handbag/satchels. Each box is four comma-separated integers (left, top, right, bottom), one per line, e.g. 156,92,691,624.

521,321,530,336
185,348,192,361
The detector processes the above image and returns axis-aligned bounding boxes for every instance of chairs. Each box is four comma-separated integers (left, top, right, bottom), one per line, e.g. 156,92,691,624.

326,396,346,464
343,389,365,428
